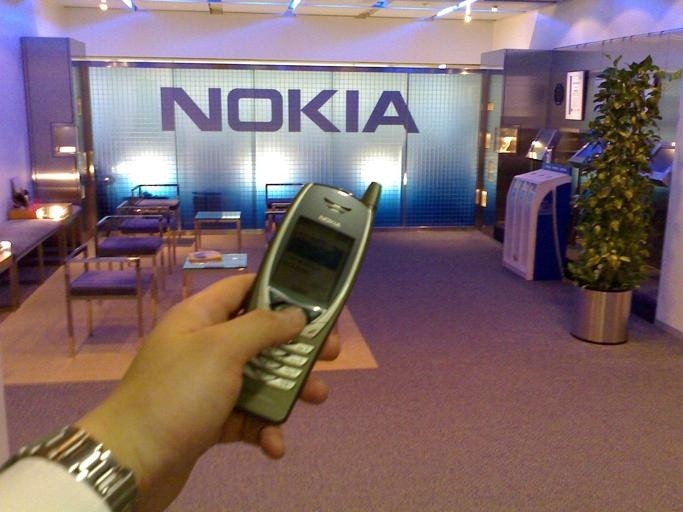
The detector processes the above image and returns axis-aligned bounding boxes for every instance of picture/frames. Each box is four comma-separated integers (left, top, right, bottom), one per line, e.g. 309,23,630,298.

565,69,589,121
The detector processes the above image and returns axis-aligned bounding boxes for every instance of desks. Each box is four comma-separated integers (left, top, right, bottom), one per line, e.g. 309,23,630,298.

181,252,250,298
194,209,244,253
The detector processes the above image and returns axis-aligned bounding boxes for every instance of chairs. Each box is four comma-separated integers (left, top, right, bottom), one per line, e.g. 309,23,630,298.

58,183,185,357
265,183,308,237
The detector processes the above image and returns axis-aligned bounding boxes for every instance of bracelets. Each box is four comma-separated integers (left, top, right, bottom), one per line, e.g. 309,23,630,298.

0,424,139,512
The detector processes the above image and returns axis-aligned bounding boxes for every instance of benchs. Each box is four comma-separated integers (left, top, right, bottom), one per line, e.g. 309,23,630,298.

0,201,82,319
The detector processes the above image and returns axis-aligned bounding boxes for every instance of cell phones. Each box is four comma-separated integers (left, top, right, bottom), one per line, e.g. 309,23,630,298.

235,180,382,426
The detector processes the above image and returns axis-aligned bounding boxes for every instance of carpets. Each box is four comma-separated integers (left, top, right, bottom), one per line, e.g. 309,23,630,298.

0,227,378,384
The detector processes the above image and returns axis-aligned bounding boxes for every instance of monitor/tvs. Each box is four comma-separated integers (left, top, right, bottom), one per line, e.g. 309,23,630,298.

525,126,561,162
638,143,676,187
568,140,610,169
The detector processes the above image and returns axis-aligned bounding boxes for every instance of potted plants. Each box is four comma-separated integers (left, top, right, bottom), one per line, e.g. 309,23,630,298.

559,45,683,345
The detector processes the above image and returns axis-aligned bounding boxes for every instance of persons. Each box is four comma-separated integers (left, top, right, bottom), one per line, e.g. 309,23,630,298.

0,273,341,512
